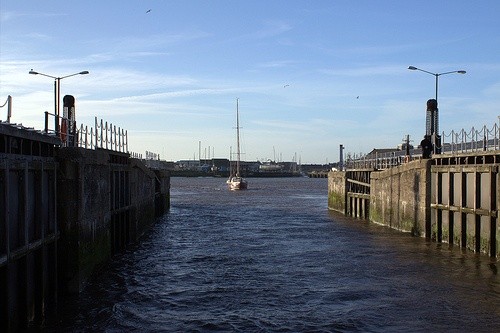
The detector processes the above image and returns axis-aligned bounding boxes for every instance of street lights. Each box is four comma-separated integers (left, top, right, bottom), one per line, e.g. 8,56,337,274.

408,65,467,133
28,68,90,137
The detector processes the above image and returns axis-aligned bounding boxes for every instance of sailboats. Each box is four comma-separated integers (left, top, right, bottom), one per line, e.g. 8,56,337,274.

227,98,248,191
